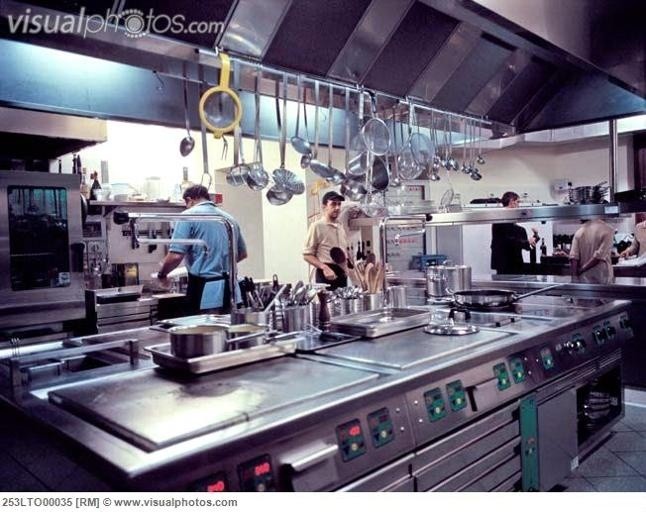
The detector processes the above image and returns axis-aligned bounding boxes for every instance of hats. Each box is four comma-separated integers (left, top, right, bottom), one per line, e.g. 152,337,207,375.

322,191,344,201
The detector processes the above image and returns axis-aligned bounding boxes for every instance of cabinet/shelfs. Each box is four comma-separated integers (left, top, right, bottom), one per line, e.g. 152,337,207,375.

344,193,646,296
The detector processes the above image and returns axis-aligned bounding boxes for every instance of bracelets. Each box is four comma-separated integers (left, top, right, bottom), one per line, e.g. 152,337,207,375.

157,272,167,279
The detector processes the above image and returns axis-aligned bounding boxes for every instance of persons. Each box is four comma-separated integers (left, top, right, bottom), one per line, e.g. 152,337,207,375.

568,219,615,285
303,190,347,289
156,185,248,316
490,191,537,275
619,220,646,258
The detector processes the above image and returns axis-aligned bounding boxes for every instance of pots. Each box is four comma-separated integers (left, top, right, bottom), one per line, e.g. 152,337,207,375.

455,283,567,307
424,260,472,300
570,178,612,205
168,322,304,355
341,92,390,202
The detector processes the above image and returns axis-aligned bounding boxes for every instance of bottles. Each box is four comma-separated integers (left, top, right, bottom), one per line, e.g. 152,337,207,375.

79,168,90,200
91,268,103,290
89,170,102,202
540,236,547,262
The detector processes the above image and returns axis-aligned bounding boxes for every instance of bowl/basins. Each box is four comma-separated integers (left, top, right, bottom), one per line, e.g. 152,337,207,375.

612,256,620,264
114,194,128,202
93,189,111,201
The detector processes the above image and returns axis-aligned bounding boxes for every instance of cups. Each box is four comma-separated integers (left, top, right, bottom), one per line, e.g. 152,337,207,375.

125,273,137,286
102,273,111,288
230,283,410,330
